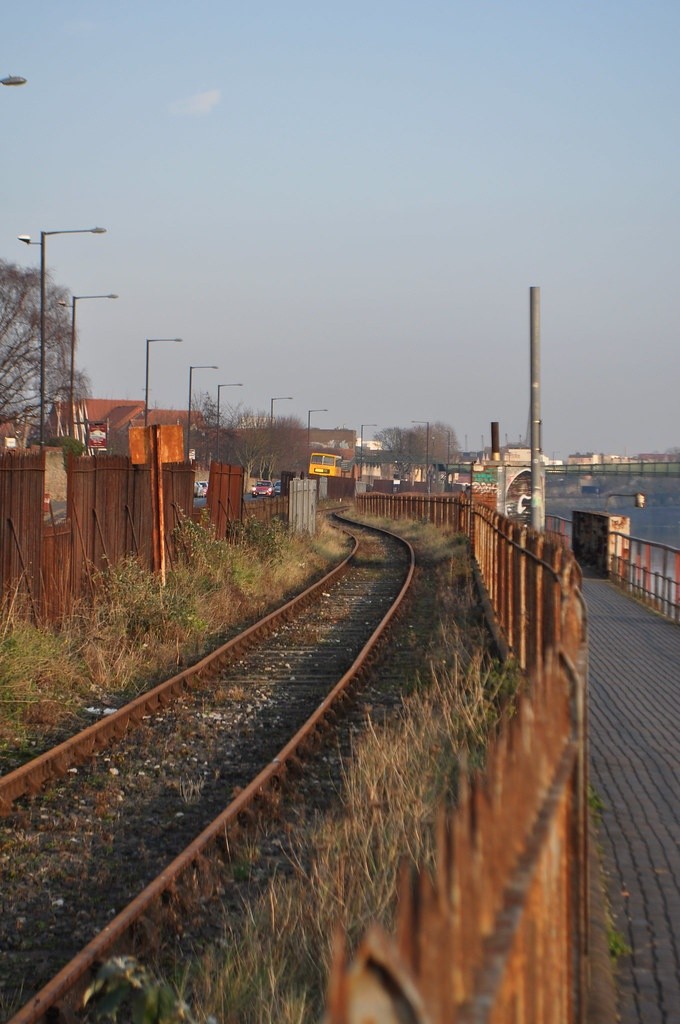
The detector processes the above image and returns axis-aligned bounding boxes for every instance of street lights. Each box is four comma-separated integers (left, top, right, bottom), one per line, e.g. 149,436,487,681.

216,383,243,461
411,420,429,484
37,226,105,450
306,409,328,478
270,397,293,431
144,335,183,425
186,365,219,459
360,423,378,482
67,293,118,440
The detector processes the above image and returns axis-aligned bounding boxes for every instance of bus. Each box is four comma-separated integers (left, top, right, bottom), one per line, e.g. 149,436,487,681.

308,451,343,479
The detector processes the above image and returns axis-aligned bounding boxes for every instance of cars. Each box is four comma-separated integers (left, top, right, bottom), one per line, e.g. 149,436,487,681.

273,480,282,494
252,481,274,497
192,480,210,498
